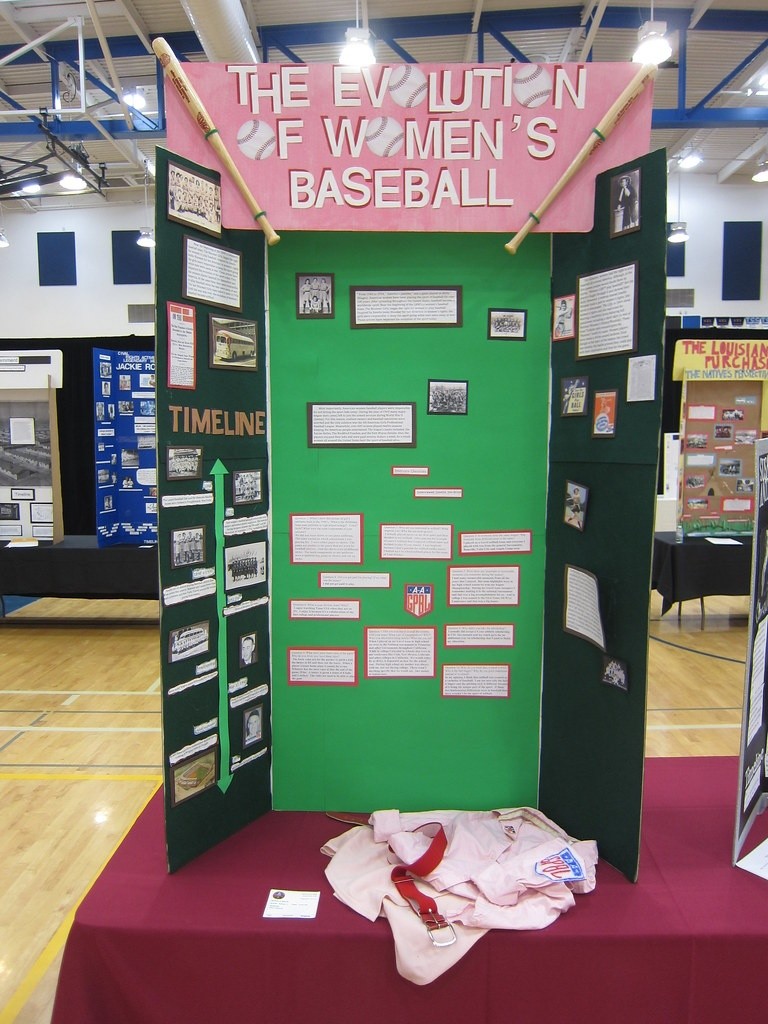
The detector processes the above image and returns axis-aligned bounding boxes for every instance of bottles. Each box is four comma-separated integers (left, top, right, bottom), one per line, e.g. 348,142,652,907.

675,526,684,543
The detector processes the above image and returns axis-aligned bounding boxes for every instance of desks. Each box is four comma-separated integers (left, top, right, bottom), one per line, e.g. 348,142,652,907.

653,530,751,632
52,756,767,1023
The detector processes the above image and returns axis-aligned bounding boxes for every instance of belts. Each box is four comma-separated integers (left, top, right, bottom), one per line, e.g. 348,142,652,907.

388,822,456,947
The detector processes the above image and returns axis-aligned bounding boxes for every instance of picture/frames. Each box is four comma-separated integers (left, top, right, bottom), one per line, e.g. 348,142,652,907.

169,747,218,808
427,378,468,415
609,166,641,239
488,308,527,339
295,272,335,318
239,631,257,668
171,525,205,569
209,311,258,373
233,470,264,506
166,446,202,480
169,620,209,664
591,388,618,438
167,159,223,239
564,480,588,531
242,704,264,750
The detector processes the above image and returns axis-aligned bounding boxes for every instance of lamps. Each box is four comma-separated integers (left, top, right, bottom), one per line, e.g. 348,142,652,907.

342,0,377,67
632,0,673,64
137,162,156,248
